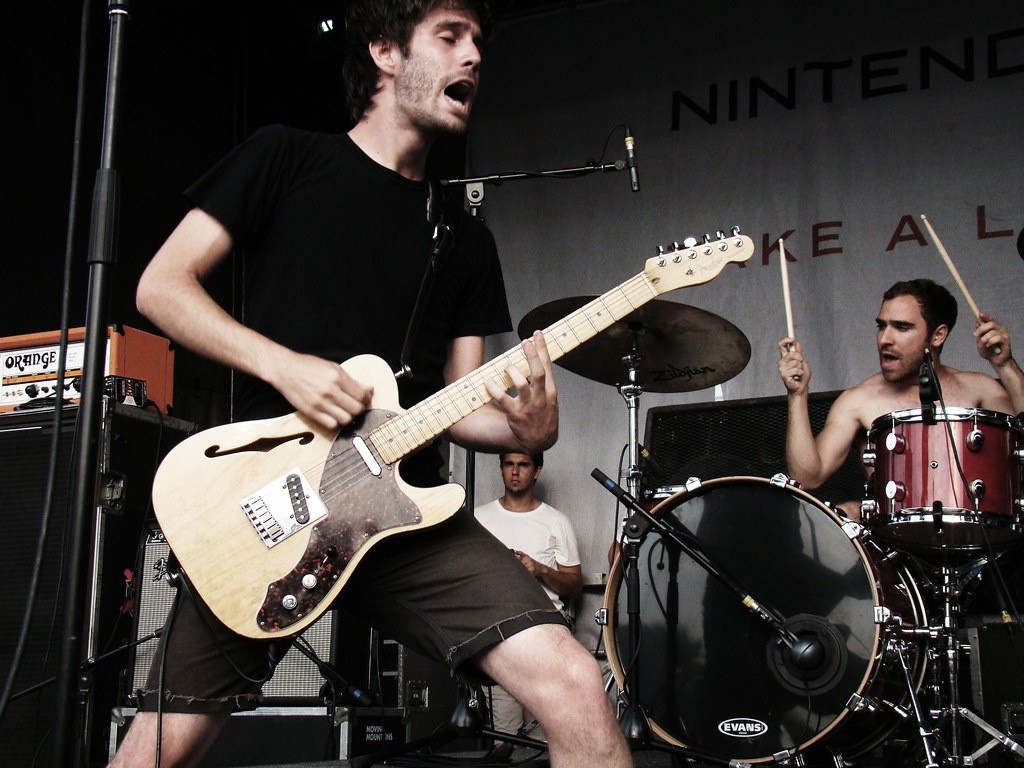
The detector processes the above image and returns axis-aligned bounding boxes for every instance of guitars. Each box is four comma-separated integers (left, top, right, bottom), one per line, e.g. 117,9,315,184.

146,223,758,668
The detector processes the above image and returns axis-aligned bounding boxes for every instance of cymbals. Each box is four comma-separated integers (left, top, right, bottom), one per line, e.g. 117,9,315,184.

514,291,755,397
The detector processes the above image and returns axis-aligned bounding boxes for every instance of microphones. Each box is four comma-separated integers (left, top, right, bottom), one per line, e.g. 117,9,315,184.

637,442,666,483
744,597,825,671
923,347,945,401
164,549,182,587
624,126,641,192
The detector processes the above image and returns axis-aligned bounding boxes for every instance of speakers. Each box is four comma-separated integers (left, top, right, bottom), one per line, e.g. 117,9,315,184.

638,389,870,518
124,525,374,708
1,406,197,767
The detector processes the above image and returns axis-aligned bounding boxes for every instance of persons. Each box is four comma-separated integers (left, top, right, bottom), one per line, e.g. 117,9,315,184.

776,280,1024,490
607,514,630,565
473,452,583,746
100,1,632,768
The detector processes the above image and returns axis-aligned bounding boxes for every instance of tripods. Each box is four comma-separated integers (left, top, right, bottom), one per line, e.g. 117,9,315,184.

347,156,639,768
908,554,1024,768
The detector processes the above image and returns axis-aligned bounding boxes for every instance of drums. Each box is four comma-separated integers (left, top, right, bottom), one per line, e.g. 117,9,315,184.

600,473,933,763
868,405,1023,552
